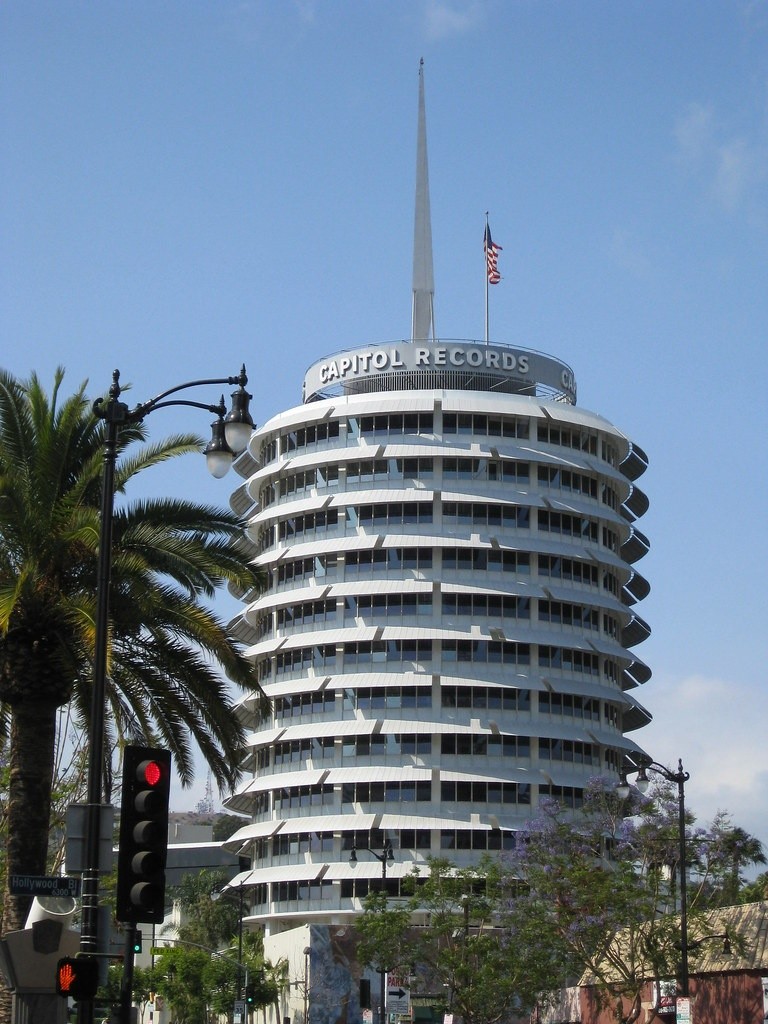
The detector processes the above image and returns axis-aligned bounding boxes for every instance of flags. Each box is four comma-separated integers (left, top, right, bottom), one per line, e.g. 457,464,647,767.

484,223,504,284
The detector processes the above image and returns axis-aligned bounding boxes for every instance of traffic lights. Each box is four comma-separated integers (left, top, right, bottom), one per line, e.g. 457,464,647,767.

133,930,142,954
117,746,172,924
246,984,254,1005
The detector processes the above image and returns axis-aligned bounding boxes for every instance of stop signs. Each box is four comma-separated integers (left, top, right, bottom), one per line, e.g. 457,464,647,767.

55,955,99,998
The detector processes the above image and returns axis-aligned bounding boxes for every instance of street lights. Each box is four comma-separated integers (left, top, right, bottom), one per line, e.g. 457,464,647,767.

617,749,733,1024
76,364,258,1024
349,838,395,1024
210,879,244,1024
